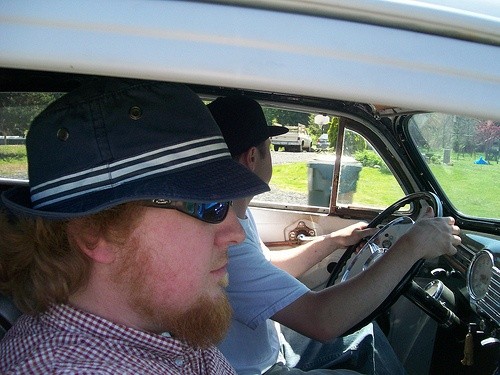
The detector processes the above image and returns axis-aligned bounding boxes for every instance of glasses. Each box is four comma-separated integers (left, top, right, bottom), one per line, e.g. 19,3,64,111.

133,198,232,224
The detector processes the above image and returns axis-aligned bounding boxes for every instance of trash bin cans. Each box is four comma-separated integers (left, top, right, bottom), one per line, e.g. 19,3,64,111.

304,156,362,206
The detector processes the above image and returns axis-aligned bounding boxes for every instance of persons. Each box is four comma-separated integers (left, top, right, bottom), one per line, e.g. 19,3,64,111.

0,77,247,375
205,94,462,375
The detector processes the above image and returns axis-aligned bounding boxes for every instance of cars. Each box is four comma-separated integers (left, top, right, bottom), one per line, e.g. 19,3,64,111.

317,133,330,149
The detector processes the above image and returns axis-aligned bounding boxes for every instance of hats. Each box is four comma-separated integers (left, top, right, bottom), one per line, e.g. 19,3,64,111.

205,95,288,141
0,79,270,221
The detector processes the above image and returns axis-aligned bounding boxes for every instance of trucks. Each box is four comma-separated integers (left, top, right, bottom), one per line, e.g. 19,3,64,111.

270,123,313,152
